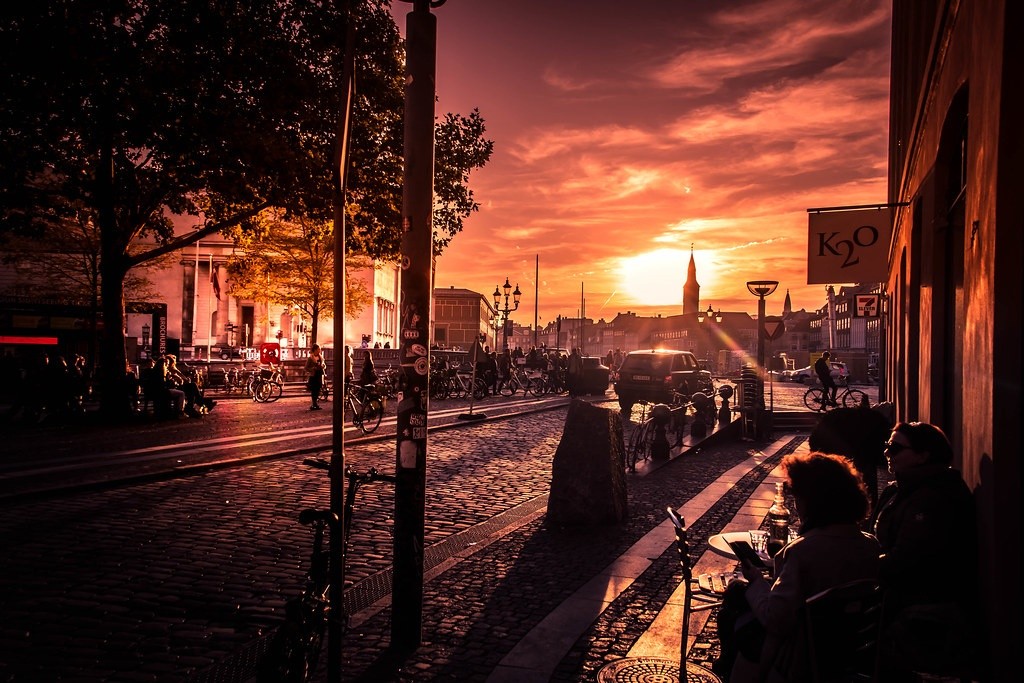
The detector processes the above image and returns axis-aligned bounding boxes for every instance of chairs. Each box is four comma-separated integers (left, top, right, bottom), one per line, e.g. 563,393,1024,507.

666,502,767,683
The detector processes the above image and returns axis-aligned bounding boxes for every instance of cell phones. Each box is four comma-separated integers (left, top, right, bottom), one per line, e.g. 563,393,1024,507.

722,537,767,570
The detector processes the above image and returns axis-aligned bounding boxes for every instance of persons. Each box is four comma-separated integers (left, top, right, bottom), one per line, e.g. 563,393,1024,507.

873,421,984,683
484,344,623,396
816,352,841,412
744,453,898,683
304,344,373,410
0,347,217,428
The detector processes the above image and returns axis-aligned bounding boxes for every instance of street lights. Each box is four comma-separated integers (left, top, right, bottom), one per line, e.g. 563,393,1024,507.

696,302,724,348
492,276,522,355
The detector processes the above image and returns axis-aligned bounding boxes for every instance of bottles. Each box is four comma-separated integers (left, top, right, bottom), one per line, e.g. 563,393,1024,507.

767,482,791,559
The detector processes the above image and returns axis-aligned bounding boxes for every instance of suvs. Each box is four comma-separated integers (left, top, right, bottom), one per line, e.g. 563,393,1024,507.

613,349,713,411
544,347,571,360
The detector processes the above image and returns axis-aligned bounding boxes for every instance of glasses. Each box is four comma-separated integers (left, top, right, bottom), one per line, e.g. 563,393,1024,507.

880,440,914,455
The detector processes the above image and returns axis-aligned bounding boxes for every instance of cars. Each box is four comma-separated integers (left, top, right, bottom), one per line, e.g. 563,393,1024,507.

779,360,850,385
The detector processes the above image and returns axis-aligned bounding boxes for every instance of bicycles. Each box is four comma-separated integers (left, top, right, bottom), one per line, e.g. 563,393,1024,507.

179,362,206,398
345,375,384,435
498,363,568,397
256,457,397,683
376,362,488,402
315,373,335,402
218,361,286,403
627,382,721,470
803,374,866,412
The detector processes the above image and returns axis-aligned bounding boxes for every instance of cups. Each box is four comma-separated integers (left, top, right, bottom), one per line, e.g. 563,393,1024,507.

749,530,768,554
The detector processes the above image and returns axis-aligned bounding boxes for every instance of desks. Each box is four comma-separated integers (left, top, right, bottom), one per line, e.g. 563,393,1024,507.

706,528,803,578
